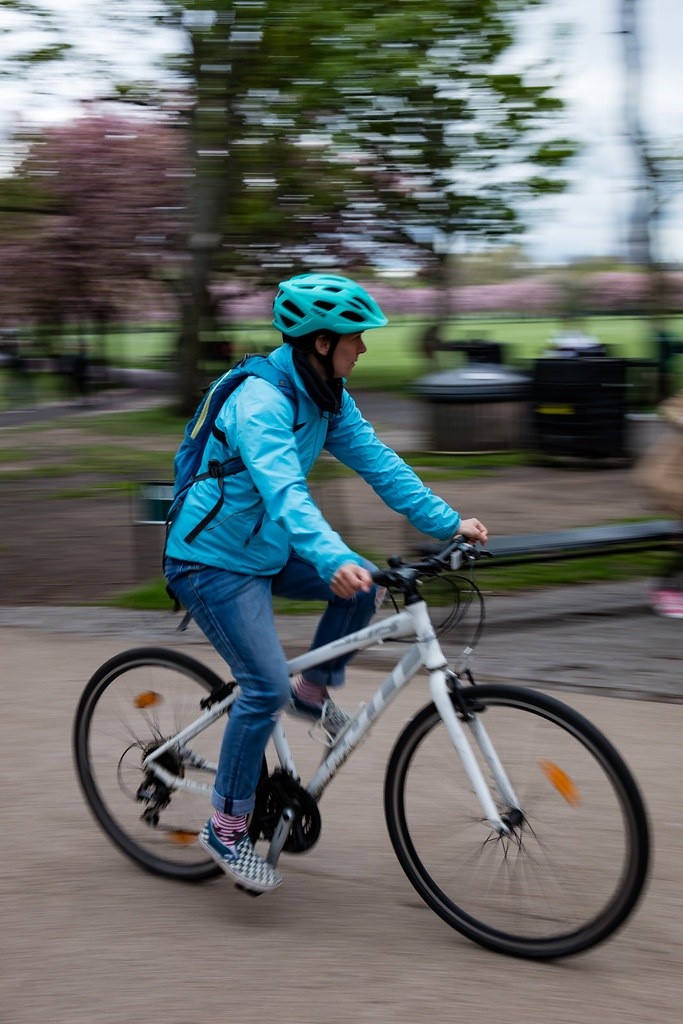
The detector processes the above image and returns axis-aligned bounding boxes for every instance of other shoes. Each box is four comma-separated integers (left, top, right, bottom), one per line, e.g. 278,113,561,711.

197,816,285,893
284,675,352,735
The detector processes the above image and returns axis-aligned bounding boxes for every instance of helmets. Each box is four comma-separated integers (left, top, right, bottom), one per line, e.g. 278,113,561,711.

267,272,391,338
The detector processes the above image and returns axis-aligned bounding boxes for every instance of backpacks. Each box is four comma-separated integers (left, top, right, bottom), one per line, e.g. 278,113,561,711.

162,350,341,615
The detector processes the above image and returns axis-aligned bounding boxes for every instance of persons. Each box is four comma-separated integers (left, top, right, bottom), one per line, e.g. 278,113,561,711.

630,391,683,619
163,275,490,892
69,341,93,406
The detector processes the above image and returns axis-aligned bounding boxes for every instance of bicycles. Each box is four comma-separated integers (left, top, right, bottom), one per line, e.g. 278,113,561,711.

73,531,650,961
